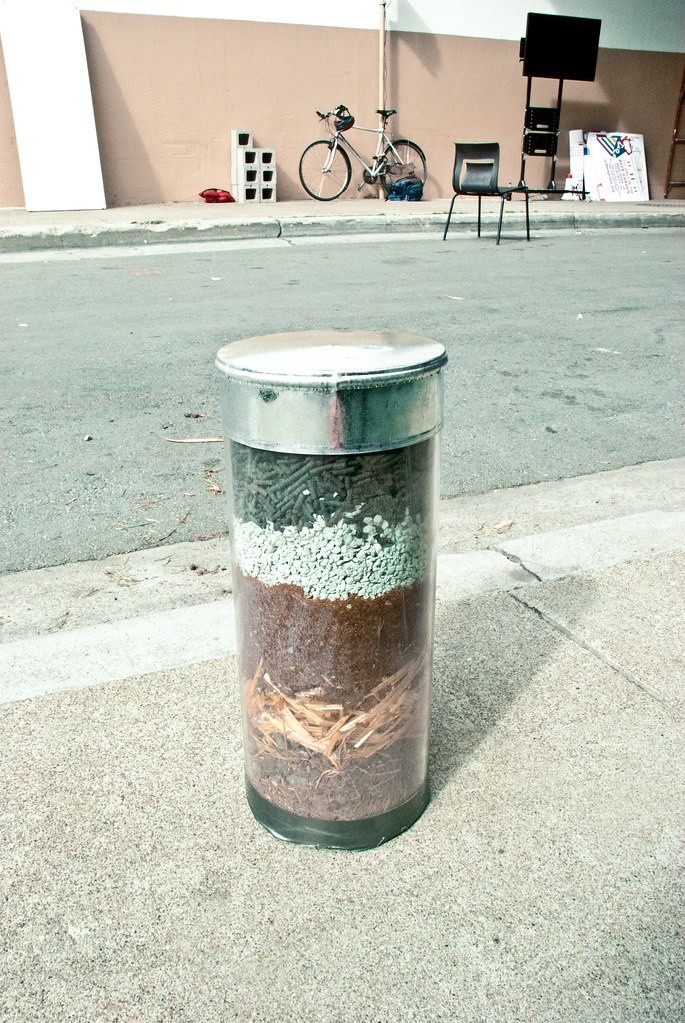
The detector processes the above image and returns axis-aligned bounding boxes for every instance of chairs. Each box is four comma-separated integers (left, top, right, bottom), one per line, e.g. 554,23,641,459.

441,142,529,247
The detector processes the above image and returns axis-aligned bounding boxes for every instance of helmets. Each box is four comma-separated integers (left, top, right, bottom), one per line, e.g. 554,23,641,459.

336,115,355,132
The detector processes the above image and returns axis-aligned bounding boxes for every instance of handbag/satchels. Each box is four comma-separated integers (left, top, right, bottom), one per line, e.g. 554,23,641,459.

388,175,423,201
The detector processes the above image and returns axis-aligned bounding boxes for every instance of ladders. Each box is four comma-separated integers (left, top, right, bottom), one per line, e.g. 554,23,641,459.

663,67,685,199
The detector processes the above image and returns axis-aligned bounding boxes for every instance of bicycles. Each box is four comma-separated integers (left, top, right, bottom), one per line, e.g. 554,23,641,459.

298,103,428,203
